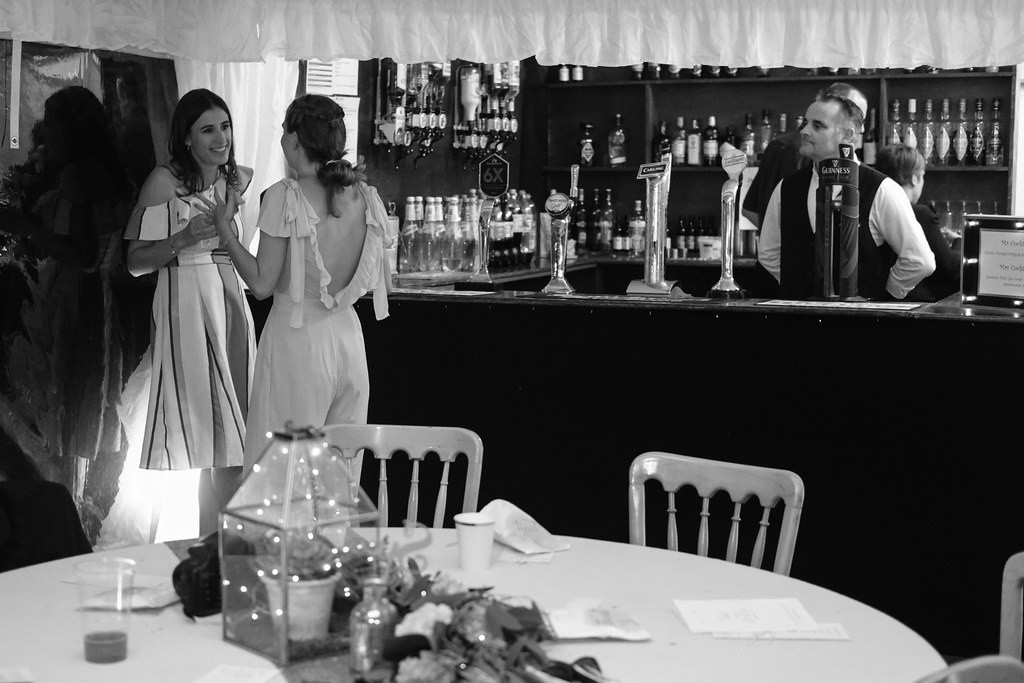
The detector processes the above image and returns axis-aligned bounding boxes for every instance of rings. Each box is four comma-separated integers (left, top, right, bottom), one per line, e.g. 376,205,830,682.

209,202,215,208
197,228,201,234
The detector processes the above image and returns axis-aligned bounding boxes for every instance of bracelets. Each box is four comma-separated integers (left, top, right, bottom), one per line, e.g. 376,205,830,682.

224,235,237,247
170,236,183,254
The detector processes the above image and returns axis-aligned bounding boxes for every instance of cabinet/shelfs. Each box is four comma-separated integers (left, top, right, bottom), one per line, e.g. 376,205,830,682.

543,64,1017,173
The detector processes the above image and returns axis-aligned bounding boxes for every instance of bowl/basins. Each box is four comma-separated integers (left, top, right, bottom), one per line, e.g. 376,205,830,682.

697,235,721,258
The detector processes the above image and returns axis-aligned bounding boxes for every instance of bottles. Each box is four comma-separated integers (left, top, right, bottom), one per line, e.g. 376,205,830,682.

630,63,740,81
580,125,594,166
399,189,537,271
864,108,877,165
387,60,521,149
349,577,400,677
557,64,584,82
888,95,1005,167
755,66,770,77
654,110,803,166
607,114,627,167
573,189,716,257
932,200,998,235
815,143,859,297
805,66,879,75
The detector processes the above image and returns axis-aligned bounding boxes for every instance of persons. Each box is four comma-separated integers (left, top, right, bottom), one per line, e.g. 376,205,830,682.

194,95,395,490
877,143,977,302
126,87,260,542
745,83,867,227
758,97,938,300
3,85,146,549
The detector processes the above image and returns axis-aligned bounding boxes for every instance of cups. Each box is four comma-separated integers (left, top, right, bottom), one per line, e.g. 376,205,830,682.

386,216,399,273
454,512,495,572
74,557,135,664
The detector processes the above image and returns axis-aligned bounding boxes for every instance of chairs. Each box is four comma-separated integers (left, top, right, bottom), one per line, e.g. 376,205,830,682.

624,451,804,575
315,421,485,529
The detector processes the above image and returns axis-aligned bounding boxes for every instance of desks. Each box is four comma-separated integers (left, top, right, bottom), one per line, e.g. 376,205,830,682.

0,525,961,683
